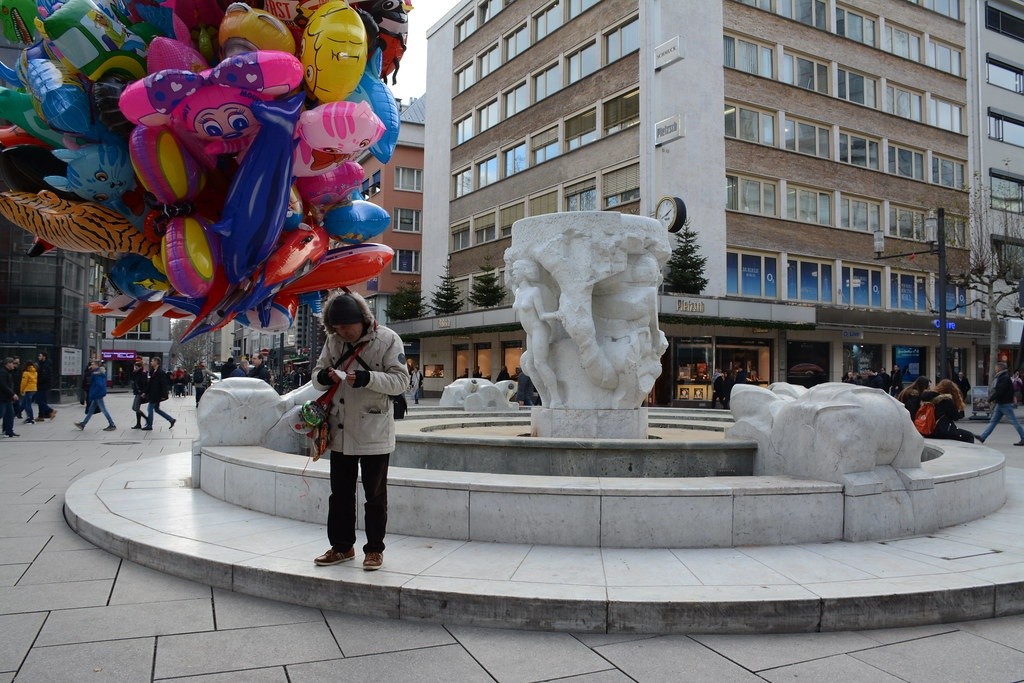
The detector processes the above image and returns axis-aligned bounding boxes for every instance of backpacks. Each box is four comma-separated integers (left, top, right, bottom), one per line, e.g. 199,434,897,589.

193,369,206,383
914,401,945,435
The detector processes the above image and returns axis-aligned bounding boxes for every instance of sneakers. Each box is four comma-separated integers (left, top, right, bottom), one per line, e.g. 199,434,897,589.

314,547,356,566
363,550,384,570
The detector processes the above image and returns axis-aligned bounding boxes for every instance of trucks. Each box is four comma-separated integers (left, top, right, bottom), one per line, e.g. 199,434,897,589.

207,361,226,383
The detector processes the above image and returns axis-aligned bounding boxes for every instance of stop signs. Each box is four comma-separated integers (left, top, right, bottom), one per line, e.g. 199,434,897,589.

262,349,269,356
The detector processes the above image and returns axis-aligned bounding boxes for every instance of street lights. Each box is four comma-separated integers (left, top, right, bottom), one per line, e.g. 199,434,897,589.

873,207,947,380
231,332,237,357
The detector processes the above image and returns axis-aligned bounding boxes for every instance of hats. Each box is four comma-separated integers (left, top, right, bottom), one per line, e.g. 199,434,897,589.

327,295,364,326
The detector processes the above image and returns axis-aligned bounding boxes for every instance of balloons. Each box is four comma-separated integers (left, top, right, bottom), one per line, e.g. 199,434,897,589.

0,0,413,345
289,400,328,460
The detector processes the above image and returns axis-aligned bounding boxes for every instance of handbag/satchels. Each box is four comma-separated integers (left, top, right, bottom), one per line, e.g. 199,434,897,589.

387,392,407,420
85,399,102,415
307,400,330,461
79,389,85,405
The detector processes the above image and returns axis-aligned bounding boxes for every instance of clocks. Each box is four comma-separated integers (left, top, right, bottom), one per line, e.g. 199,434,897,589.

654,196,687,234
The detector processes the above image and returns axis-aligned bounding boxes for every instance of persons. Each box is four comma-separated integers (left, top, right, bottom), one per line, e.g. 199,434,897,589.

515,372,533,406
409,367,423,404
220,353,274,388
897,376,974,444
311,292,409,571
954,372,972,401
166,366,191,397
132,357,175,430
290,367,310,388
496,366,509,382
973,362,1024,446
195,362,219,407
505,260,565,410
841,364,904,397
712,367,747,409
473,366,483,378
74,359,116,431
0,351,57,437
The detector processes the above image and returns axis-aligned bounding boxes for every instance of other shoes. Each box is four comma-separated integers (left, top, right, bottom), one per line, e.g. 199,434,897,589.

3,430,20,438
145,417,148,425
974,434,985,443
131,424,141,428
74,422,84,431
49,408,57,421
168,419,176,429
142,426,153,431
34,416,44,422
25,418,34,424
103,424,116,431
16,410,23,419
1013,439,1024,446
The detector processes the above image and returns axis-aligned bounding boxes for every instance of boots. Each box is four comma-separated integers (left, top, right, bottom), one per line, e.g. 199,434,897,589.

414,398,419,404
